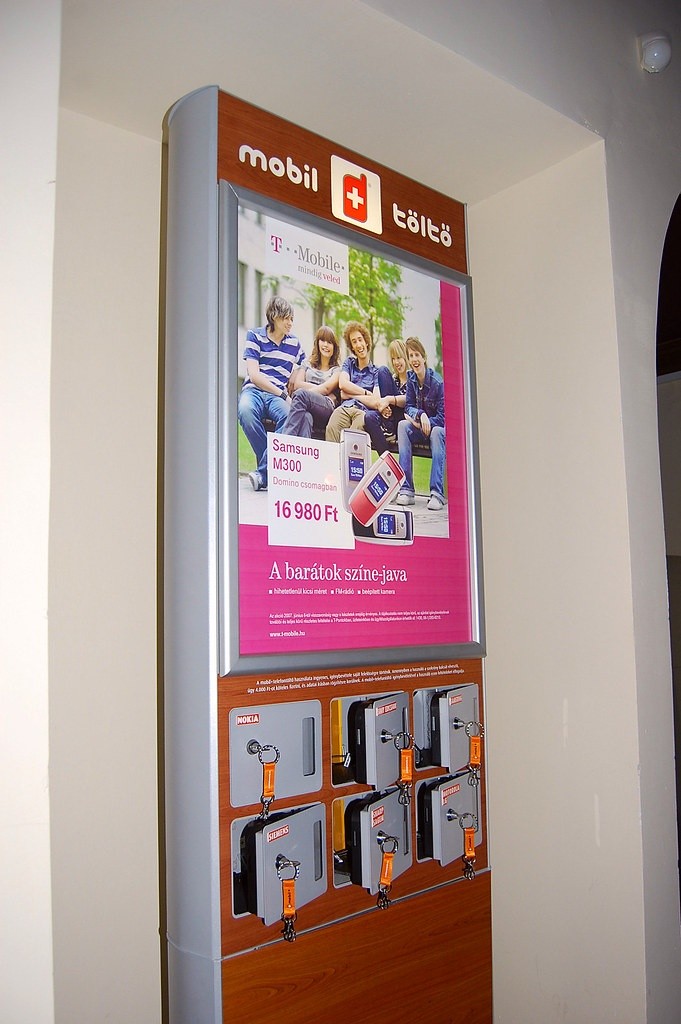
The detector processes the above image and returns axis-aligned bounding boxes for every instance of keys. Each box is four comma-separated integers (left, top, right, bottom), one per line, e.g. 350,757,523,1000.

392,734,401,738
458,814,467,818
388,836,399,841
464,723,472,726
257,745,270,752
284,861,301,867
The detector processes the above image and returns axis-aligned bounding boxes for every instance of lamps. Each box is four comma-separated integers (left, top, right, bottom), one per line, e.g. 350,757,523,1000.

636,29,673,74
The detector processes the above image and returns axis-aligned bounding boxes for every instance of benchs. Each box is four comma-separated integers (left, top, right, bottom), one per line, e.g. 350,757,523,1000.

260,417,433,459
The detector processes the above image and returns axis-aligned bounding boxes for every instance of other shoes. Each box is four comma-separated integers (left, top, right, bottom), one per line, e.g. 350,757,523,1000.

427,496,444,510
397,494,415,505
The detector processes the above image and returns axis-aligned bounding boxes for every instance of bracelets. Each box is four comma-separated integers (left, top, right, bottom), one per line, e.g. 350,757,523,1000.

280,392,287,400
394,396,397,406
365,390,367,395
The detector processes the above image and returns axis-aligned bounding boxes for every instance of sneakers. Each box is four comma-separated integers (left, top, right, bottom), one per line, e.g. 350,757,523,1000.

249,472,264,490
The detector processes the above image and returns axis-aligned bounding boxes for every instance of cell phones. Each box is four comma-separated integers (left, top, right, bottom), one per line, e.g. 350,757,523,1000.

341,426,413,545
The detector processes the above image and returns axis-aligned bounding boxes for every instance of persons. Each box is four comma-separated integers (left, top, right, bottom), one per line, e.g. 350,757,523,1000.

364,339,412,457
398,337,446,510
283,326,342,438
326,322,380,443
238,296,306,490
